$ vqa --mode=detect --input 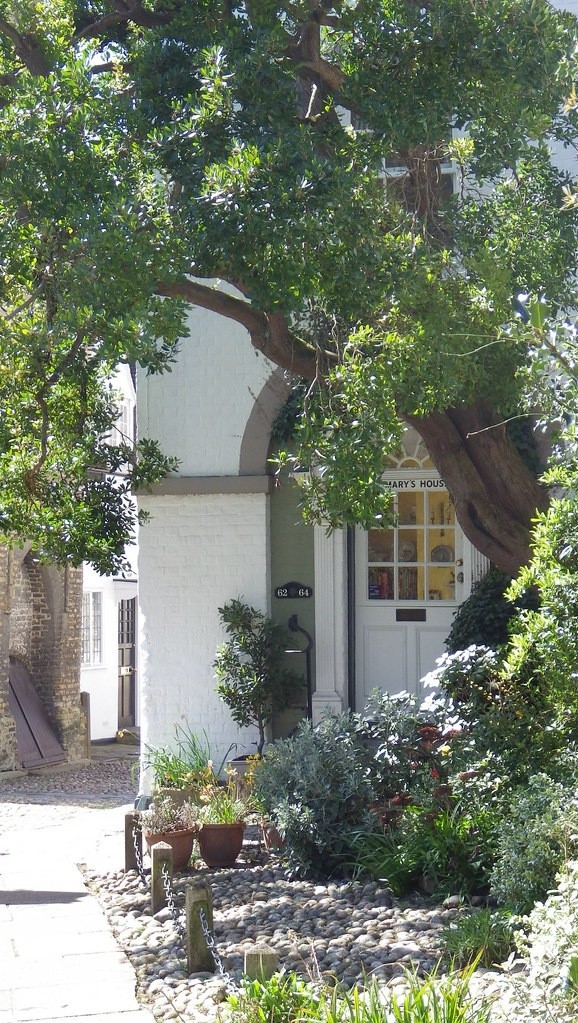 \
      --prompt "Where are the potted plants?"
[137,792,196,870]
[196,793,246,870]
[117,713,210,806]
[211,593,305,789]
